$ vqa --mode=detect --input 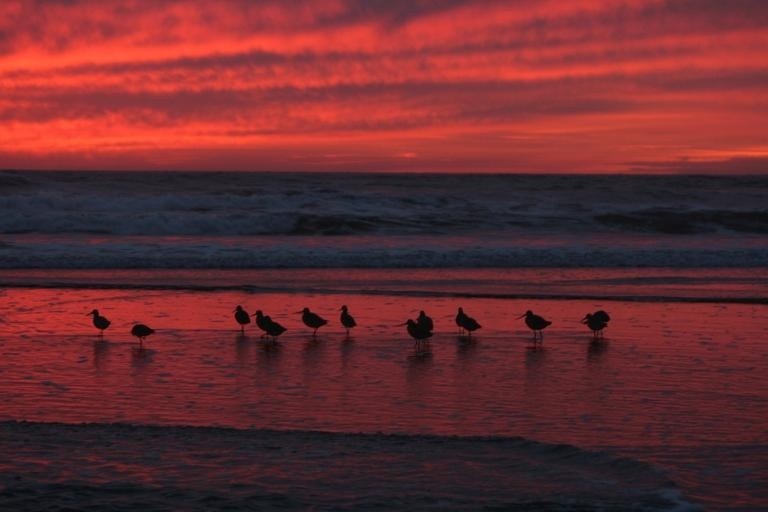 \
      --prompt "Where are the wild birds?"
[516,309,553,339]
[86,309,112,338]
[402,310,433,348]
[131,323,155,344]
[455,307,482,336]
[581,310,611,337]
[336,303,357,335]
[251,309,288,346]
[292,307,330,338]
[230,304,252,332]
